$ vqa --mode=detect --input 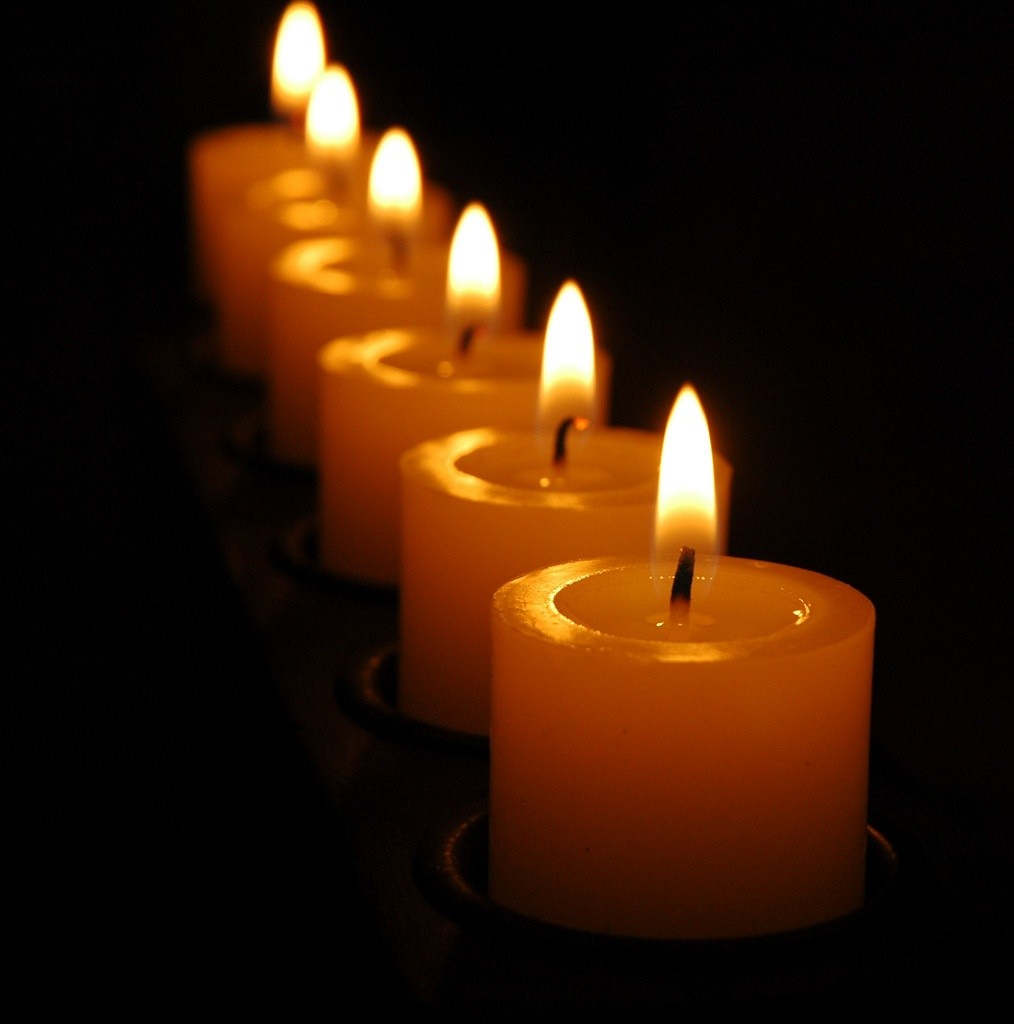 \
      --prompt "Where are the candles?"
[269,128,529,463]
[320,202,616,587]
[192,0,457,366]
[485,381,878,942]
[400,280,735,736]
